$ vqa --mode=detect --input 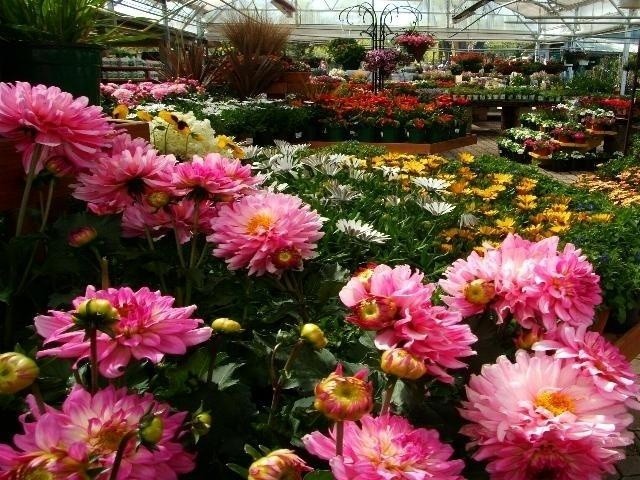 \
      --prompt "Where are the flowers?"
[0,28,640,480]
